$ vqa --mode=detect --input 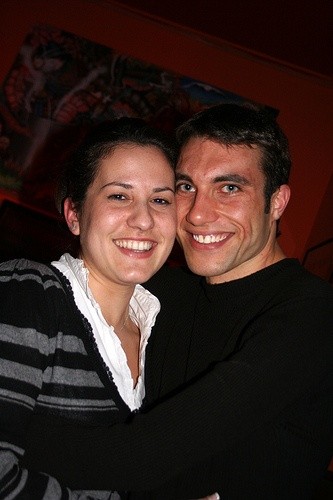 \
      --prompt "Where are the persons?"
[0,117,180,500]
[147,104,333,500]
